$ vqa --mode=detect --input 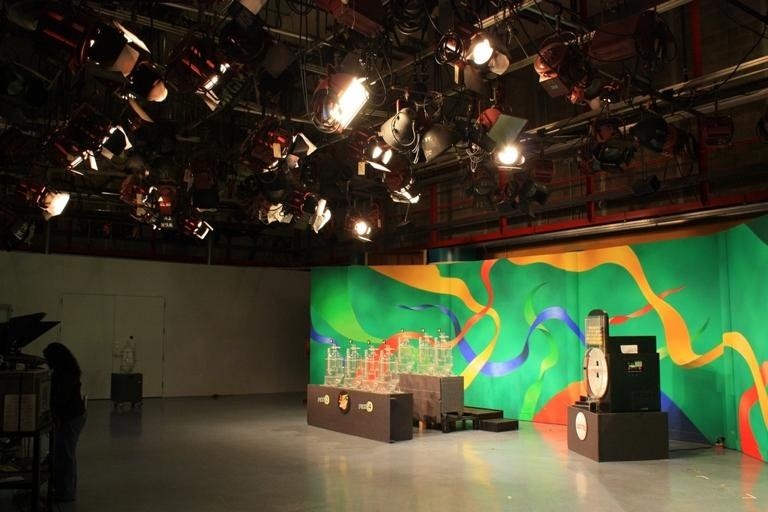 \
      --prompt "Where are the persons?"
[40,343,86,503]
